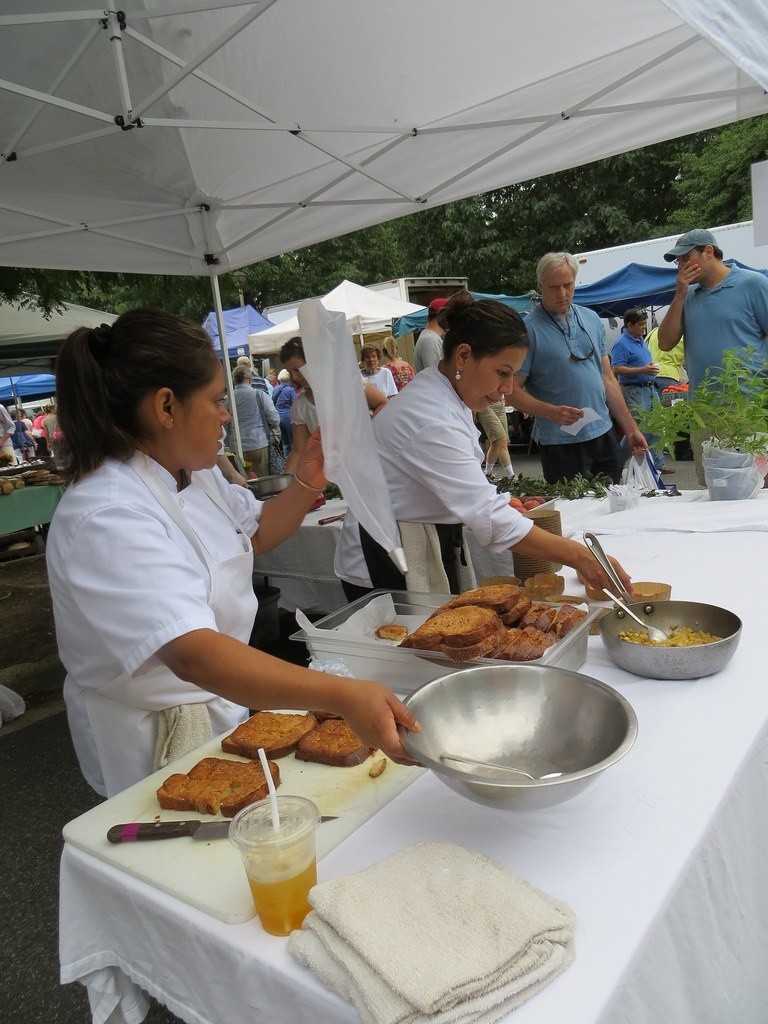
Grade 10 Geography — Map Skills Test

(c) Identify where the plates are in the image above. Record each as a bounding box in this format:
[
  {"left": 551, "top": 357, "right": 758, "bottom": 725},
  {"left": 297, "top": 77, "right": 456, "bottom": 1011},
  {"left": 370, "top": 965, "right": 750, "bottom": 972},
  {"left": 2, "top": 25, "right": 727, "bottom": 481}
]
[{"left": 509, "top": 509, "right": 564, "bottom": 583}]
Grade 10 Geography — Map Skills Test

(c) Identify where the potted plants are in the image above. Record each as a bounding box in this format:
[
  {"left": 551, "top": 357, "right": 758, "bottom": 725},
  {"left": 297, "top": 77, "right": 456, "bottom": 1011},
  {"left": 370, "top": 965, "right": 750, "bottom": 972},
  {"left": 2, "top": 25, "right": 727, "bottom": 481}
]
[{"left": 632, "top": 339, "right": 768, "bottom": 501}]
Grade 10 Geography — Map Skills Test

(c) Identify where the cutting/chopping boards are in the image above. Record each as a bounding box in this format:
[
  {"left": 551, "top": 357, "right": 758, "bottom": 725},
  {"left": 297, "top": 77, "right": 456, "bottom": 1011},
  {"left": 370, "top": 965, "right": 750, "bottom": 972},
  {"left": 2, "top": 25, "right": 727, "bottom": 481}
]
[{"left": 61, "top": 710, "right": 428, "bottom": 925}]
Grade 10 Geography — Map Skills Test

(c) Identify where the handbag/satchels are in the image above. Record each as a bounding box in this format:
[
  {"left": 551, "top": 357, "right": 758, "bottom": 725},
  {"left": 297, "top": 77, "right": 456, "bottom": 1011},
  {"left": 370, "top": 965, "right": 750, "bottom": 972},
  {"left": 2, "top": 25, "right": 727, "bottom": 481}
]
[
  {"left": 17, "top": 432, "right": 34, "bottom": 446},
  {"left": 31, "top": 429, "right": 43, "bottom": 439},
  {"left": 54, "top": 429, "right": 63, "bottom": 442},
  {"left": 626, "top": 450, "right": 667, "bottom": 490},
  {"left": 269, "top": 442, "right": 287, "bottom": 476}
]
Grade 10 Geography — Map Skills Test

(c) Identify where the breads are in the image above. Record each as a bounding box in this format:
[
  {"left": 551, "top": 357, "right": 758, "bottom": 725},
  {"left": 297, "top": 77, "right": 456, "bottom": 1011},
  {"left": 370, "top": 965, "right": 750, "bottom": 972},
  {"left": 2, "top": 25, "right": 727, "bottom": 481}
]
[
  {"left": 156, "top": 709, "right": 388, "bottom": 818},
  {"left": 0, "top": 469, "right": 64, "bottom": 494},
  {"left": 376, "top": 584, "right": 588, "bottom": 662}
]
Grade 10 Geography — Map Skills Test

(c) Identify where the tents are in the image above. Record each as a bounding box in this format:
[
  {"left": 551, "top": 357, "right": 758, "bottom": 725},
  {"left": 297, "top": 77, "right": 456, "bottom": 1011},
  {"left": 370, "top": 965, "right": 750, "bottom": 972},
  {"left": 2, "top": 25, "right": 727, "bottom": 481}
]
[
  {"left": 247, "top": 279, "right": 428, "bottom": 373},
  {"left": 531, "top": 259, "right": 768, "bottom": 342},
  {"left": 391, "top": 289, "right": 547, "bottom": 339},
  {"left": 199, "top": 303, "right": 276, "bottom": 362},
  {"left": 0, "top": 289, "right": 125, "bottom": 462}
]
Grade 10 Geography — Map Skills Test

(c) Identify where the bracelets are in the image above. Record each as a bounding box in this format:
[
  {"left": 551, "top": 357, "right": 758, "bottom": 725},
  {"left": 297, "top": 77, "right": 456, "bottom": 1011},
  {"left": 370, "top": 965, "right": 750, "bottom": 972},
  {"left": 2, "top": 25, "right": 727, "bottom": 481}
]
[{"left": 293, "top": 473, "right": 328, "bottom": 492}]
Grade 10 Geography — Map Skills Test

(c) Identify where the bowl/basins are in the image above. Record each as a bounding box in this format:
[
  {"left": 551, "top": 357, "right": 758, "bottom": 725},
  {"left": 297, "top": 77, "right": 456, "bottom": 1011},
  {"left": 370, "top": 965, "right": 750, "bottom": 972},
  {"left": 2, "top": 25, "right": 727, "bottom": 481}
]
[
  {"left": 480, "top": 573, "right": 622, "bottom": 636},
  {"left": 397, "top": 663, "right": 640, "bottom": 809},
  {"left": 623, "top": 581, "right": 671, "bottom": 601}
]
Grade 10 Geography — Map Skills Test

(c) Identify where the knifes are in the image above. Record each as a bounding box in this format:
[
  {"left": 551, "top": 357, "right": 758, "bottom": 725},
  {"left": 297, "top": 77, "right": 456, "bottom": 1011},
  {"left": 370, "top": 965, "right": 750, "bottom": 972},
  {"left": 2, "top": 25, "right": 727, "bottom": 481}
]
[{"left": 106, "top": 816, "right": 339, "bottom": 841}]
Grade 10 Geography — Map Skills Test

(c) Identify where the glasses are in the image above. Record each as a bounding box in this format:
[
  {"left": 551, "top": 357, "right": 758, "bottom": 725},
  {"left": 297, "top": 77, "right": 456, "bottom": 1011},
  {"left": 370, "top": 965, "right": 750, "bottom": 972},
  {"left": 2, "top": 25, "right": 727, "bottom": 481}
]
[
  {"left": 564, "top": 328, "right": 595, "bottom": 362},
  {"left": 636, "top": 308, "right": 647, "bottom": 317},
  {"left": 278, "top": 341, "right": 300, "bottom": 352},
  {"left": 673, "top": 249, "right": 696, "bottom": 265}
]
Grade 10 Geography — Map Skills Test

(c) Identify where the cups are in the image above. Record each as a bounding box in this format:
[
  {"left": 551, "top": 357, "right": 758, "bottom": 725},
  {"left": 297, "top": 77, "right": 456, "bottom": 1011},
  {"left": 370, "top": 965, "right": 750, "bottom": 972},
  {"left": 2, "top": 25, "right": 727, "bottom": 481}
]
[
  {"left": 607, "top": 491, "right": 640, "bottom": 512},
  {"left": 227, "top": 795, "right": 321, "bottom": 937}
]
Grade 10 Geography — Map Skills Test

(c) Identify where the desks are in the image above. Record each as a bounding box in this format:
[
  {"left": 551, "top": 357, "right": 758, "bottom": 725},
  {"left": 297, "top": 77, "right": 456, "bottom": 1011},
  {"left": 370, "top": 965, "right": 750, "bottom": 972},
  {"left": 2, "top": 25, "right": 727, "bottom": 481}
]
[{"left": 61, "top": 489, "right": 768, "bottom": 1024}]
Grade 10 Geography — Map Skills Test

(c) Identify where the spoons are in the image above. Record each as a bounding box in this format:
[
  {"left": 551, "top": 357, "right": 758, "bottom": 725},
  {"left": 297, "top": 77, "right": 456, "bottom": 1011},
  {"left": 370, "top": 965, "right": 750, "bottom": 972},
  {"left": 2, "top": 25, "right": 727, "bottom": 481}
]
[{"left": 441, "top": 753, "right": 572, "bottom": 782}]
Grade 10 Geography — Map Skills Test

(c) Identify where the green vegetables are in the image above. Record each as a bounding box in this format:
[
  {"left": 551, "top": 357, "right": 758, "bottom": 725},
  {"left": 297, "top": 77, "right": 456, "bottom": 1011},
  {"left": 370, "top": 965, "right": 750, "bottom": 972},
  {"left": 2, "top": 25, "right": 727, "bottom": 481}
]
[
  {"left": 640, "top": 487, "right": 683, "bottom": 498},
  {"left": 322, "top": 482, "right": 344, "bottom": 500},
  {"left": 497, "top": 473, "right": 607, "bottom": 500}
]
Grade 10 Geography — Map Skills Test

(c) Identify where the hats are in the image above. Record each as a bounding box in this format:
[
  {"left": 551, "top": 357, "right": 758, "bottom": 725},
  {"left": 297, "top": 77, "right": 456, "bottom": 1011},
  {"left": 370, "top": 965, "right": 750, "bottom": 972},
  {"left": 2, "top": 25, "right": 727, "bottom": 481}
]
[
  {"left": 428, "top": 298, "right": 449, "bottom": 314},
  {"left": 663, "top": 229, "right": 723, "bottom": 263}
]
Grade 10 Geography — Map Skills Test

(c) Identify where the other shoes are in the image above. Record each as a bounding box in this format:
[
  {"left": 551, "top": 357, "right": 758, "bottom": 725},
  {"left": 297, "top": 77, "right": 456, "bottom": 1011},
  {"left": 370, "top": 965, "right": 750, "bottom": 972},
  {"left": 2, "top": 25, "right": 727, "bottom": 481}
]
[
  {"left": 0, "top": 591, "right": 11, "bottom": 600},
  {"left": 481, "top": 461, "right": 486, "bottom": 467},
  {"left": 659, "top": 465, "right": 676, "bottom": 474},
  {"left": 485, "top": 469, "right": 502, "bottom": 482}
]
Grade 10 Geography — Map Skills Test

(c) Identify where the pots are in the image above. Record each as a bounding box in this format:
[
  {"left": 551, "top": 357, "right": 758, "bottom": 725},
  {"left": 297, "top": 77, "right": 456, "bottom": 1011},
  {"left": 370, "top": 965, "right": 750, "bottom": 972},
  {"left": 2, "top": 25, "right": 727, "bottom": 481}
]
[
  {"left": 246, "top": 472, "right": 293, "bottom": 500},
  {"left": 583, "top": 531, "right": 743, "bottom": 680}
]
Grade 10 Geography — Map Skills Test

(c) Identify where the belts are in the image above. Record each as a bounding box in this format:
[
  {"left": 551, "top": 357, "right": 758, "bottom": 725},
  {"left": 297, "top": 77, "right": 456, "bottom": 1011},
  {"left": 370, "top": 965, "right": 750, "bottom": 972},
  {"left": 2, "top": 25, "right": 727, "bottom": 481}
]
[{"left": 623, "top": 382, "right": 653, "bottom": 388}]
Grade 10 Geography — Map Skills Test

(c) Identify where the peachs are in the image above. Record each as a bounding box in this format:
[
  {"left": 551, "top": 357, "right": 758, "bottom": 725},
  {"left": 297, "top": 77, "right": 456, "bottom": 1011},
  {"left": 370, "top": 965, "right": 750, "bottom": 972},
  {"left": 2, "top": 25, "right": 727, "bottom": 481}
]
[{"left": 509, "top": 496, "right": 546, "bottom": 512}]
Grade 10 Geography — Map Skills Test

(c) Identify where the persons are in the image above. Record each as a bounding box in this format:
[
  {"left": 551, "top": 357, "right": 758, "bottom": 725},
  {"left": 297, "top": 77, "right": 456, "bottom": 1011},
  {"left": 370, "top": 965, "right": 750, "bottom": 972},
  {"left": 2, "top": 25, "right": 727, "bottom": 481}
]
[
  {"left": 45, "top": 306, "right": 430, "bottom": 809},
  {"left": 656, "top": 229, "right": 768, "bottom": 488},
  {"left": 608, "top": 308, "right": 690, "bottom": 473},
  {"left": 218, "top": 355, "right": 297, "bottom": 490},
  {"left": 0, "top": 402, "right": 62, "bottom": 468},
  {"left": 332, "top": 288, "right": 638, "bottom": 613},
  {"left": 277, "top": 335, "right": 389, "bottom": 501},
  {"left": 359, "top": 298, "right": 519, "bottom": 485},
  {"left": 503, "top": 251, "right": 649, "bottom": 489}
]
